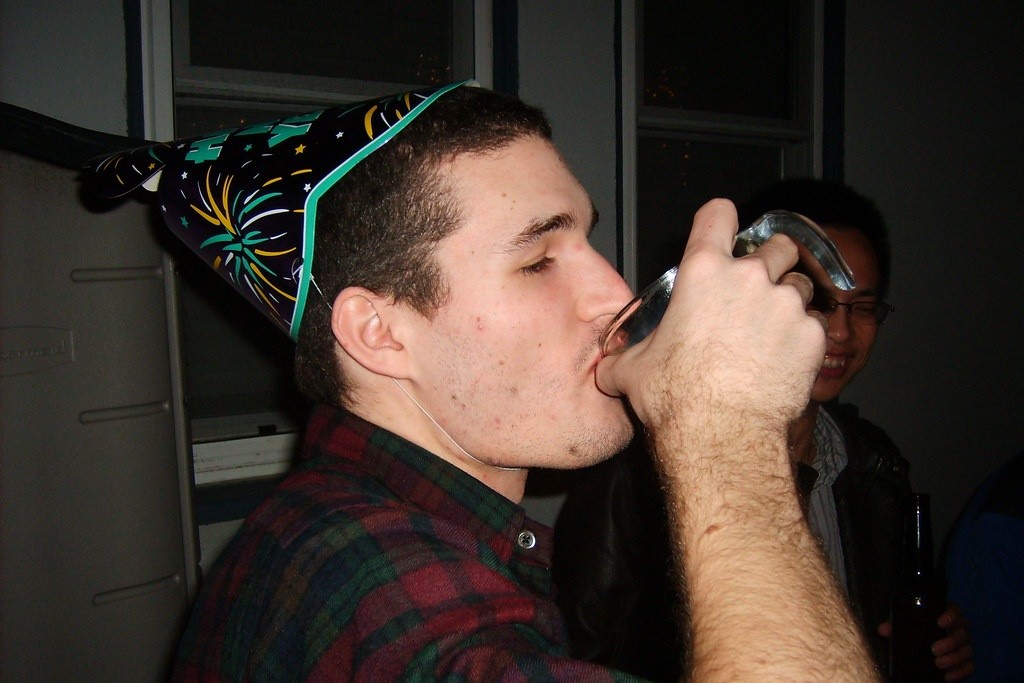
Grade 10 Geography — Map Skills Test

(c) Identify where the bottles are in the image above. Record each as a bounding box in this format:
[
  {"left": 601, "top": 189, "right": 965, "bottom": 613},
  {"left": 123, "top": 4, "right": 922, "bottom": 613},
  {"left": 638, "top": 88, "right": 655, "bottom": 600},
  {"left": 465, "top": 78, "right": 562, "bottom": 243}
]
[{"left": 890, "top": 493, "right": 948, "bottom": 683}]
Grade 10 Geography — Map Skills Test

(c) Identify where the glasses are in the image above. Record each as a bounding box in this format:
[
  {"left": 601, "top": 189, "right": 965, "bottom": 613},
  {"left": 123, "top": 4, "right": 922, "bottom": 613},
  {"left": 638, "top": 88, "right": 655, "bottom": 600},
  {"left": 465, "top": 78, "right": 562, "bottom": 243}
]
[{"left": 805, "top": 299, "right": 897, "bottom": 326}]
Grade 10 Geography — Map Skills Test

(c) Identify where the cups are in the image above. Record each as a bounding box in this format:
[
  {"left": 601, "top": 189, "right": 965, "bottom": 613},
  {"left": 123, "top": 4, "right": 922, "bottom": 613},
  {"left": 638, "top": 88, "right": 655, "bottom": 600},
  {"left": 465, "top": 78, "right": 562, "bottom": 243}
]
[{"left": 599, "top": 210, "right": 856, "bottom": 357}]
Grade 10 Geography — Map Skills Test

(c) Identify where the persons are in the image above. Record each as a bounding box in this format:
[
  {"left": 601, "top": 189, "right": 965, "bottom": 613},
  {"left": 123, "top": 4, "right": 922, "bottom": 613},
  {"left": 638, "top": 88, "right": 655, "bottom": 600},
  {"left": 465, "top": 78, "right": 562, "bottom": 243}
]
[
  {"left": 554, "top": 174, "right": 974, "bottom": 683},
  {"left": 81, "top": 77, "right": 882, "bottom": 683}
]
